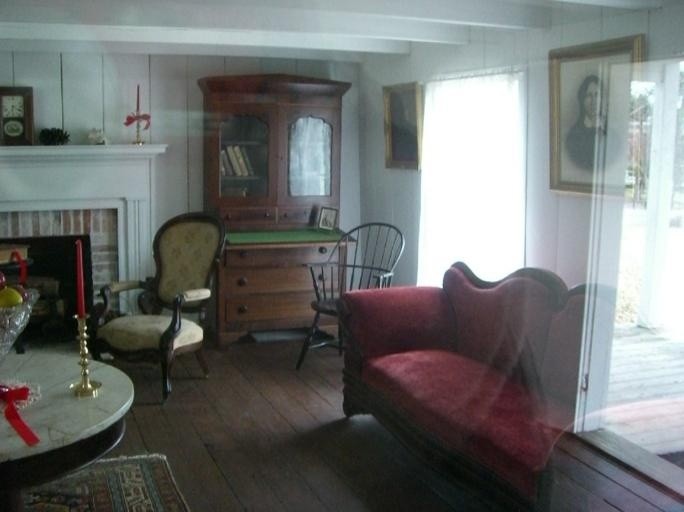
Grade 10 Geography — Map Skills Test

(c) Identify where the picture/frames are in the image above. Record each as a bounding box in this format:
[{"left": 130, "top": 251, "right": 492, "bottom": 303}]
[
  {"left": 382, "top": 82, "right": 420, "bottom": 168},
  {"left": 318, "top": 206, "right": 339, "bottom": 232},
  {"left": 549, "top": 34, "right": 647, "bottom": 200}
]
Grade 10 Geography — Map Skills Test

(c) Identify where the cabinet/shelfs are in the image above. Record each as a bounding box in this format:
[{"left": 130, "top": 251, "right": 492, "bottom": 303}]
[
  {"left": 196, "top": 72, "right": 352, "bottom": 231},
  {"left": 206, "top": 231, "right": 356, "bottom": 344}
]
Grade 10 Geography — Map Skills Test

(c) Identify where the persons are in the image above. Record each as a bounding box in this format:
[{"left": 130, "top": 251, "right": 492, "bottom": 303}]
[{"left": 563, "top": 74, "right": 624, "bottom": 173}]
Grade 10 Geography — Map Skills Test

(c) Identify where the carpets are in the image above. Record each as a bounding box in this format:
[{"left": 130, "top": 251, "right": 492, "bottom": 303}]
[{"left": 0, "top": 452, "right": 189, "bottom": 512}]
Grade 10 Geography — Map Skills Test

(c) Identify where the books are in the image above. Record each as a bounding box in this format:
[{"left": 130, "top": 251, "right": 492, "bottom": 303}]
[
  {"left": 219, "top": 145, "right": 255, "bottom": 198},
  {"left": 1, "top": 242, "right": 30, "bottom": 265}
]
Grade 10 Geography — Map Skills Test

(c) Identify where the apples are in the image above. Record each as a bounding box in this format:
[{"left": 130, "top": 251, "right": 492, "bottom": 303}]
[
  {"left": 0, "top": 287, "right": 23, "bottom": 307},
  {"left": 0, "top": 272, "right": 6, "bottom": 290},
  {"left": 9, "top": 284, "right": 29, "bottom": 302}
]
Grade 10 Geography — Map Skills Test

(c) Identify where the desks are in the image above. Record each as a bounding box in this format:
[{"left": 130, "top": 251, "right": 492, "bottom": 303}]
[{"left": 0, "top": 351, "right": 134, "bottom": 512}]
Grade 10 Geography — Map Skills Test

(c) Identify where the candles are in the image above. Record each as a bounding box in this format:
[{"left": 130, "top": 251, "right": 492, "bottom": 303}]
[
  {"left": 137, "top": 85, "right": 140, "bottom": 110},
  {"left": 75, "top": 240, "right": 86, "bottom": 317}
]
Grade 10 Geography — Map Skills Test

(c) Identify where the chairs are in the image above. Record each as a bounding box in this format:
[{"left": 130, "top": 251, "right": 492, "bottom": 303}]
[
  {"left": 88, "top": 212, "right": 227, "bottom": 405},
  {"left": 296, "top": 221, "right": 406, "bottom": 370}
]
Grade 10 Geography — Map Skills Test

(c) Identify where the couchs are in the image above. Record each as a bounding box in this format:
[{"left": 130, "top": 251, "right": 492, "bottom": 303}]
[{"left": 337, "top": 263, "right": 617, "bottom": 512}]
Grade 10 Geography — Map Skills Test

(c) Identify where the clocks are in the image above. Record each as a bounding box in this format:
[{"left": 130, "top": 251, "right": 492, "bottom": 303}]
[{"left": 0, "top": 86, "right": 33, "bottom": 146}]
